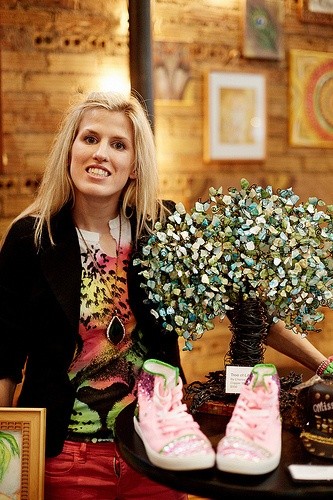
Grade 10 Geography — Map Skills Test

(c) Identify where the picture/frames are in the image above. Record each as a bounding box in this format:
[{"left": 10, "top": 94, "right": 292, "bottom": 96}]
[
  {"left": 202, "top": 67, "right": 268, "bottom": 166},
  {"left": 0, "top": 409, "right": 46, "bottom": 500},
  {"left": 242, "top": 1, "right": 286, "bottom": 64},
  {"left": 289, "top": 47, "right": 333, "bottom": 150},
  {"left": 295, "top": 0, "right": 333, "bottom": 27}
]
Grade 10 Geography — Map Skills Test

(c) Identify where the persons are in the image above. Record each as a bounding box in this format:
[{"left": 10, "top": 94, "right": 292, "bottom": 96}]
[{"left": 1, "top": 92, "right": 333, "bottom": 500}]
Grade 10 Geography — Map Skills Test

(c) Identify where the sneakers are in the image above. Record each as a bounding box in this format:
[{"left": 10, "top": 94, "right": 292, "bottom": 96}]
[
  {"left": 216, "top": 363, "right": 281, "bottom": 475},
  {"left": 133, "top": 359, "right": 216, "bottom": 470}
]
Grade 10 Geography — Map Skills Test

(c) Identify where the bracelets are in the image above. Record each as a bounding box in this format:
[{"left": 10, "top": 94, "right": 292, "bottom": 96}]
[{"left": 316, "top": 355, "right": 333, "bottom": 381}]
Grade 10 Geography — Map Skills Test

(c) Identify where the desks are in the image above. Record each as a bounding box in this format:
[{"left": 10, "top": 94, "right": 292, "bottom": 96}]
[{"left": 111, "top": 377, "right": 333, "bottom": 500}]
